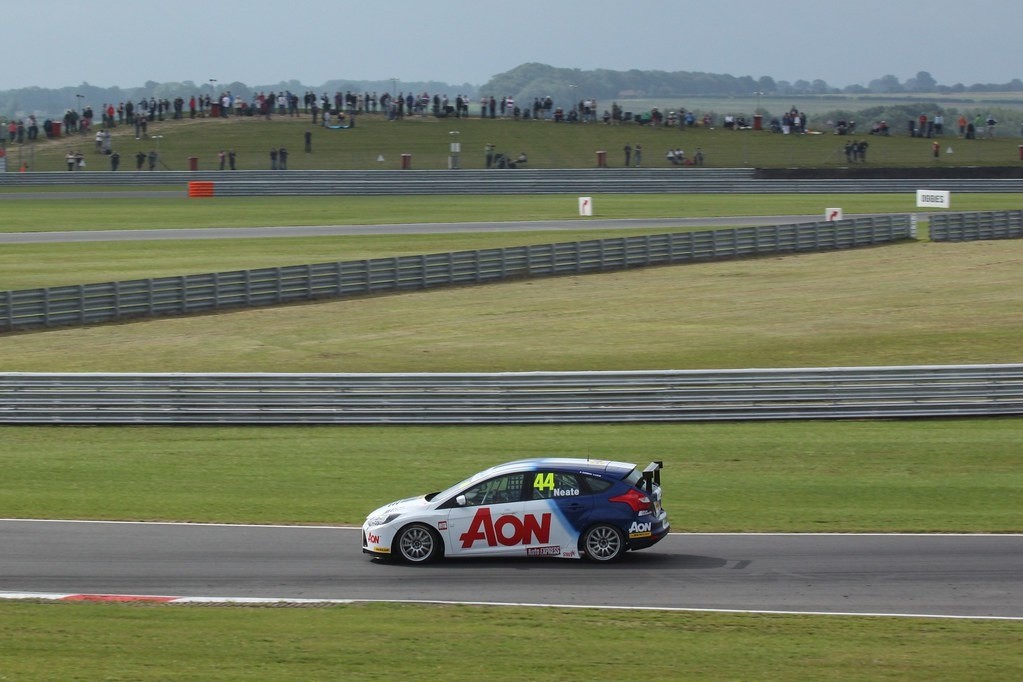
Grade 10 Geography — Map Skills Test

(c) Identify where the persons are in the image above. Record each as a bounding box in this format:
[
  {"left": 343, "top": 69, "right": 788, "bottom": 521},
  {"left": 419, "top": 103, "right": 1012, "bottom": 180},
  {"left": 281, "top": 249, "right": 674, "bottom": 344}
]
[{"left": 0, "top": 90, "right": 998, "bottom": 171}]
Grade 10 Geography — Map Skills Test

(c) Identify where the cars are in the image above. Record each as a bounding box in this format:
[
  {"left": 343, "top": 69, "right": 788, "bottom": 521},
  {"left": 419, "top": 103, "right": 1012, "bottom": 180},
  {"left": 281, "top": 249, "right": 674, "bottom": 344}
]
[{"left": 360, "top": 457, "right": 672, "bottom": 566}]
